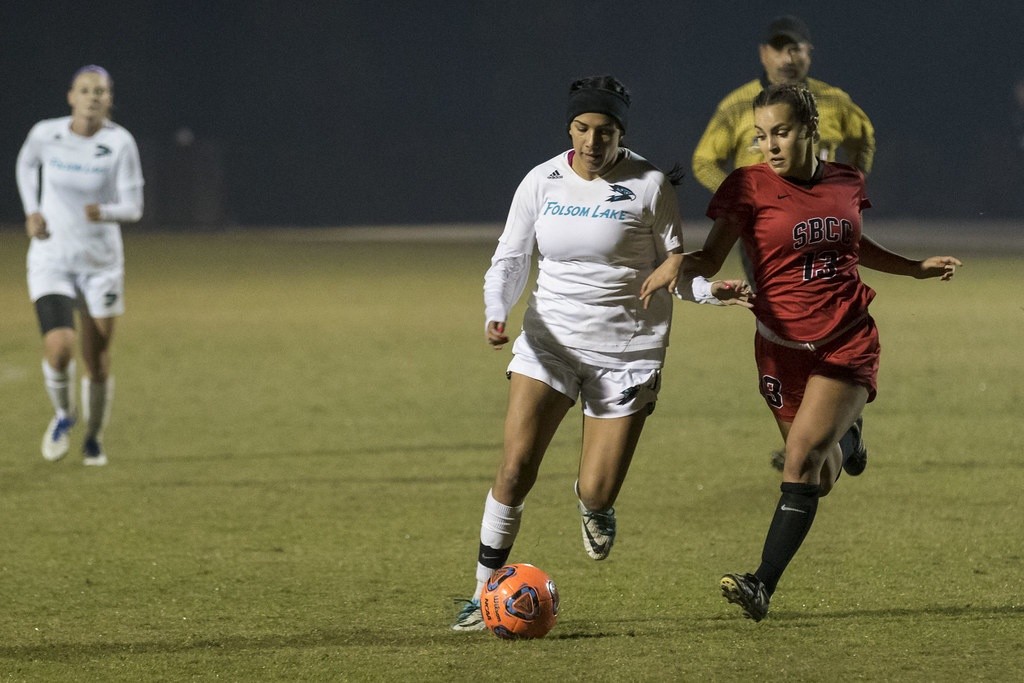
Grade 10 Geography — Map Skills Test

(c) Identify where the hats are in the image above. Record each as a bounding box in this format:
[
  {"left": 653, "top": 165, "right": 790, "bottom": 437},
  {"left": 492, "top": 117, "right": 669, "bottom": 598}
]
[
  {"left": 566, "top": 74, "right": 632, "bottom": 136},
  {"left": 761, "top": 14, "right": 811, "bottom": 44}
]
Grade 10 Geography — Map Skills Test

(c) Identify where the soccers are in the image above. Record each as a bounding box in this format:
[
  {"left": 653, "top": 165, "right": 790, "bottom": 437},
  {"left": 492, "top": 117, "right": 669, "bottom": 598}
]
[{"left": 480, "top": 563, "right": 560, "bottom": 639}]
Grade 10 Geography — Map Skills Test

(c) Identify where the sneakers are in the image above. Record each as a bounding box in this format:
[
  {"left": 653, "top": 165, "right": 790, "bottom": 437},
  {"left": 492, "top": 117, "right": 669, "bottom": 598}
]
[
  {"left": 720, "top": 572, "right": 770, "bottom": 623},
  {"left": 842, "top": 415, "right": 867, "bottom": 476},
  {"left": 574, "top": 479, "right": 616, "bottom": 560},
  {"left": 451, "top": 597, "right": 487, "bottom": 632},
  {"left": 40, "top": 406, "right": 77, "bottom": 462},
  {"left": 83, "top": 438, "right": 108, "bottom": 466}
]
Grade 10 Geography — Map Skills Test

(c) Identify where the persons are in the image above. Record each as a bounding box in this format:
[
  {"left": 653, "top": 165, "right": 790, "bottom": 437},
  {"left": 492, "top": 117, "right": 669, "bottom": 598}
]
[
  {"left": 452, "top": 75, "right": 756, "bottom": 631},
  {"left": 691, "top": 14, "right": 875, "bottom": 470},
  {"left": 15, "top": 66, "right": 144, "bottom": 466},
  {"left": 638, "top": 83, "right": 963, "bottom": 621}
]
